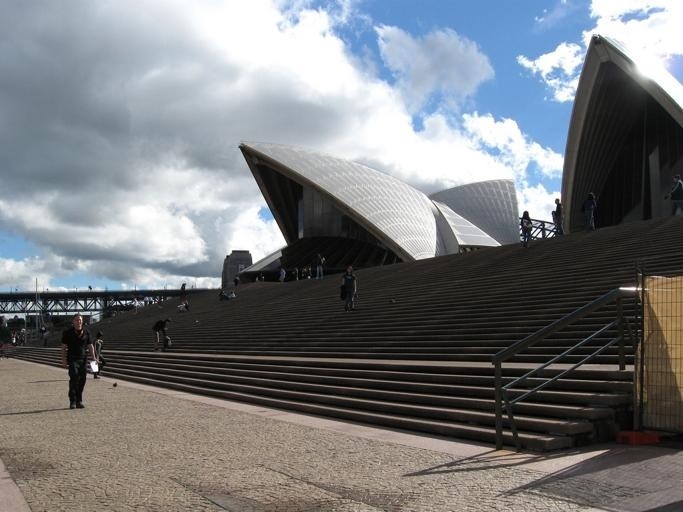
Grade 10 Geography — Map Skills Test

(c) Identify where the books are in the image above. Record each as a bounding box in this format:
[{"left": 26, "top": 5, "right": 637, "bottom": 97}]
[{"left": 90, "top": 360, "right": 99, "bottom": 372}]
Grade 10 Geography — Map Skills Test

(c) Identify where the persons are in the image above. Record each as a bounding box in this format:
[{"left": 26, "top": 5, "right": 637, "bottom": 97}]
[
  {"left": 0, "top": 283, "right": 190, "bottom": 382},
  {"left": 582, "top": 192, "right": 595, "bottom": 226},
  {"left": 217, "top": 254, "right": 324, "bottom": 302},
  {"left": 551, "top": 211, "right": 558, "bottom": 224},
  {"left": 553, "top": 198, "right": 560, "bottom": 234},
  {"left": 520, "top": 210, "right": 532, "bottom": 245},
  {"left": 340, "top": 266, "right": 358, "bottom": 312},
  {"left": 60, "top": 314, "right": 102, "bottom": 410},
  {"left": 663, "top": 174, "right": 682, "bottom": 214}
]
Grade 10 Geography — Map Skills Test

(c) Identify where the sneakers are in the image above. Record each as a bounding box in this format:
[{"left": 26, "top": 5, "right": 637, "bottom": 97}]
[
  {"left": 344, "top": 307, "right": 348, "bottom": 312},
  {"left": 350, "top": 308, "right": 358, "bottom": 313},
  {"left": 93, "top": 372, "right": 103, "bottom": 379}
]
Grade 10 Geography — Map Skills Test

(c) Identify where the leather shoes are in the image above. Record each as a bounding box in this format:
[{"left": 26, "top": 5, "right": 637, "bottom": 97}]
[
  {"left": 77, "top": 401, "right": 85, "bottom": 408},
  {"left": 70, "top": 401, "right": 76, "bottom": 409}
]
